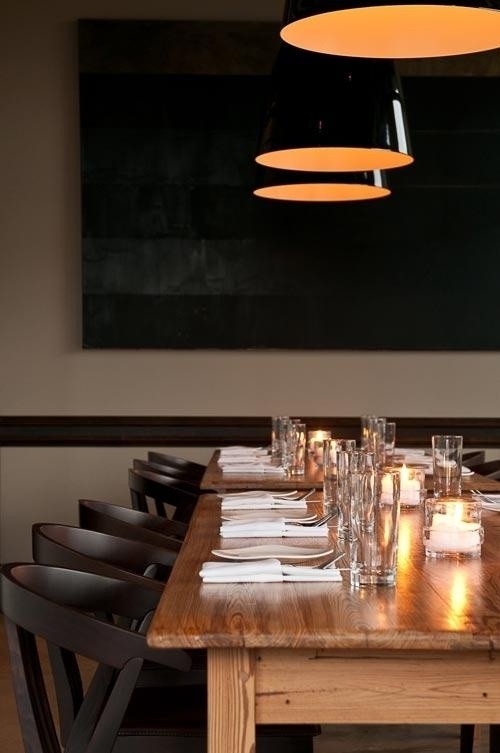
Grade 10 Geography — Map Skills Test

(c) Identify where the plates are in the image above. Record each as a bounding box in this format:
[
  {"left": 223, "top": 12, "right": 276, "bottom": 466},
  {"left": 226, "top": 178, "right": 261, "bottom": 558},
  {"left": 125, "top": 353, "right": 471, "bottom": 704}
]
[
  {"left": 215, "top": 490, "right": 300, "bottom": 499},
  {"left": 219, "top": 511, "right": 319, "bottom": 522},
  {"left": 209, "top": 544, "right": 335, "bottom": 562}
]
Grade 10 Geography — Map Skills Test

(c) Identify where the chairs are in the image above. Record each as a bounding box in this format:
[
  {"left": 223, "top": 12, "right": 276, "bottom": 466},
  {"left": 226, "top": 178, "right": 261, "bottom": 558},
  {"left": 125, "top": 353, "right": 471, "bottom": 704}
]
[{"left": 462, "top": 450, "right": 500, "bottom": 482}]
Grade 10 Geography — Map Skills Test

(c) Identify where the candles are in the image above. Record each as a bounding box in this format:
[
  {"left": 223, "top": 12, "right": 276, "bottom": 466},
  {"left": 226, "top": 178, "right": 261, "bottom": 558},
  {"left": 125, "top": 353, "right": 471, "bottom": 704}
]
[
  {"left": 383, "top": 479, "right": 420, "bottom": 506},
  {"left": 430, "top": 514, "right": 480, "bottom": 554}
]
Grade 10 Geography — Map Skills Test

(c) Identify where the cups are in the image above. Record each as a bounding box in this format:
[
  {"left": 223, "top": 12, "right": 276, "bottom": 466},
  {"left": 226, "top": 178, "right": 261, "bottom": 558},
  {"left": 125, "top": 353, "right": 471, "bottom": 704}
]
[
  {"left": 320, "top": 438, "right": 402, "bottom": 588},
  {"left": 270, "top": 414, "right": 308, "bottom": 478},
  {"left": 361, "top": 412, "right": 397, "bottom": 473},
  {"left": 431, "top": 434, "right": 463, "bottom": 500}
]
